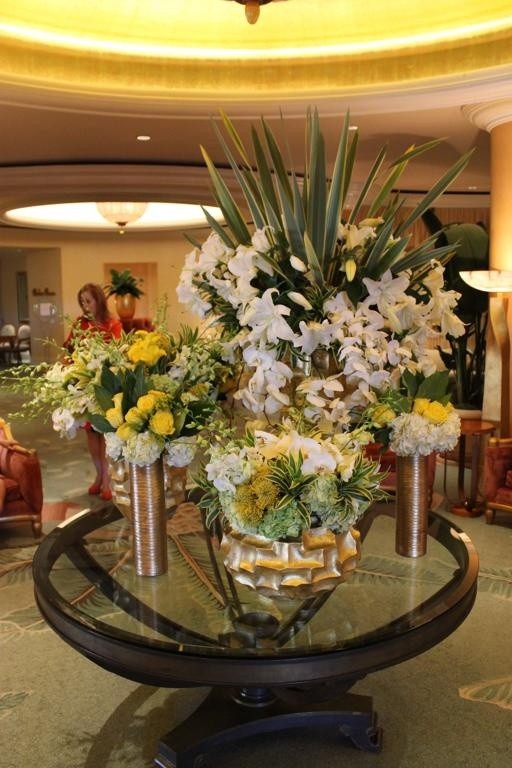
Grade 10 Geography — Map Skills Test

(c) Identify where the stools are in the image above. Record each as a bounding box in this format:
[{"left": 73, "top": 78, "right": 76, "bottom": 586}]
[{"left": 443, "top": 422, "right": 495, "bottom": 517}]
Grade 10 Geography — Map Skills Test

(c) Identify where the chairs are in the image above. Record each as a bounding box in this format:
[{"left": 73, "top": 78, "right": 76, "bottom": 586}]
[
  {"left": 0, "top": 418, "right": 43, "bottom": 540},
  {"left": 484, "top": 436, "right": 512, "bottom": 524}
]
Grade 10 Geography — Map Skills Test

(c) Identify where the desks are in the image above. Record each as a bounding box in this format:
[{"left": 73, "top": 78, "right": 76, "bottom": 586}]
[{"left": 32, "top": 483, "right": 481, "bottom": 768}]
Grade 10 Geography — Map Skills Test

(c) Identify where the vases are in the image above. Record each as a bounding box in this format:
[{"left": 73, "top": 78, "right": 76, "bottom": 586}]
[
  {"left": 395, "top": 453, "right": 431, "bottom": 558},
  {"left": 105, "top": 456, "right": 187, "bottom": 525},
  {"left": 217, "top": 527, "right": 360, "bottom": 601},
  {"left": 124, "top": 462, "right": 167, "bottom": 577}
]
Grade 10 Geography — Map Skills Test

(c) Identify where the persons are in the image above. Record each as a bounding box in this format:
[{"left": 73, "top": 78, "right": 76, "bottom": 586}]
[{"left": 58, "top": 282, "right": 124, "bottom": 501}]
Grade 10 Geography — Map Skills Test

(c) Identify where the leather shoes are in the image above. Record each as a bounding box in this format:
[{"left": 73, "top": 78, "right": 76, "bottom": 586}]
[
  {"left": 88, "top": 483, "right": 102, "bottom": 493},
  {"left": 100, "top": 486, "right": 112, "bottom": 500}
]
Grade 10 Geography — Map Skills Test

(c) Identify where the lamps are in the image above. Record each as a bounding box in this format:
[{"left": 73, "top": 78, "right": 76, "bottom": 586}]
[{"left": 95, "top": 200, "right": 147, "bottom": 231}]
